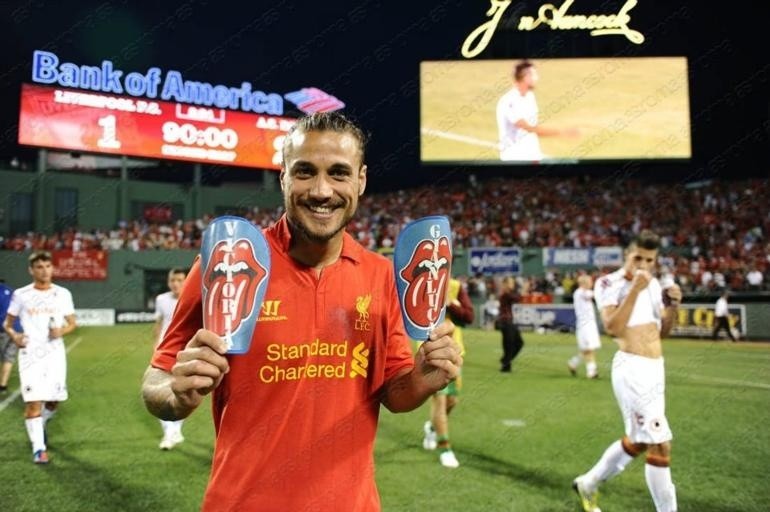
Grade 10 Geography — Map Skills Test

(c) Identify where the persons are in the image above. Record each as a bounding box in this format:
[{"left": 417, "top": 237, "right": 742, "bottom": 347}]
[
  {"left": 491, "top": 61, "right": 582, "bottom": 160},
  {"left": 565, "top": 273, "right": 606, "bottom": 378},
  {"left": 569, "top": 228, "right": 684, "bottom": 512},
  {"left": 492, "top": 273, "right": 532, "bottom": 373},
  {"left": 343, "top": 173, "right": 767, "bottom": 342},
  {"left": 142, "top": 110, "right": 466, "bottom": 512},
  {"left": 2, "top": 248, "right": 78, "bottom": 463},
  {"left": 0, "top": 206, "right": 286, "bottom": 251},
  {"left": 0, "top": 279, "right": 22, "bottom": 396},
  {"left": 416, "top": 275, "right": 472, "bottom": 472},
  {"left": 152, "top": 264, "right": 189, "bottom": 452}
]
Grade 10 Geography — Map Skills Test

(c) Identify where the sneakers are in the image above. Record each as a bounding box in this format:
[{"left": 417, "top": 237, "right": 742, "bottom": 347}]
[
  {"left": 440, "top": 451, "right": 460, "bottom": 468},
  {"left": 572, "top": 476, "right": 602, "bottom": 512},
  {"left": 159, "top": 434, "right": 176, "bottom": 450},
  {"left": 32, "top": 449, "right": 48, "bottom": 464},
  {"left": 423, "top": 420, "right": 438, "bottom": 450}
]
